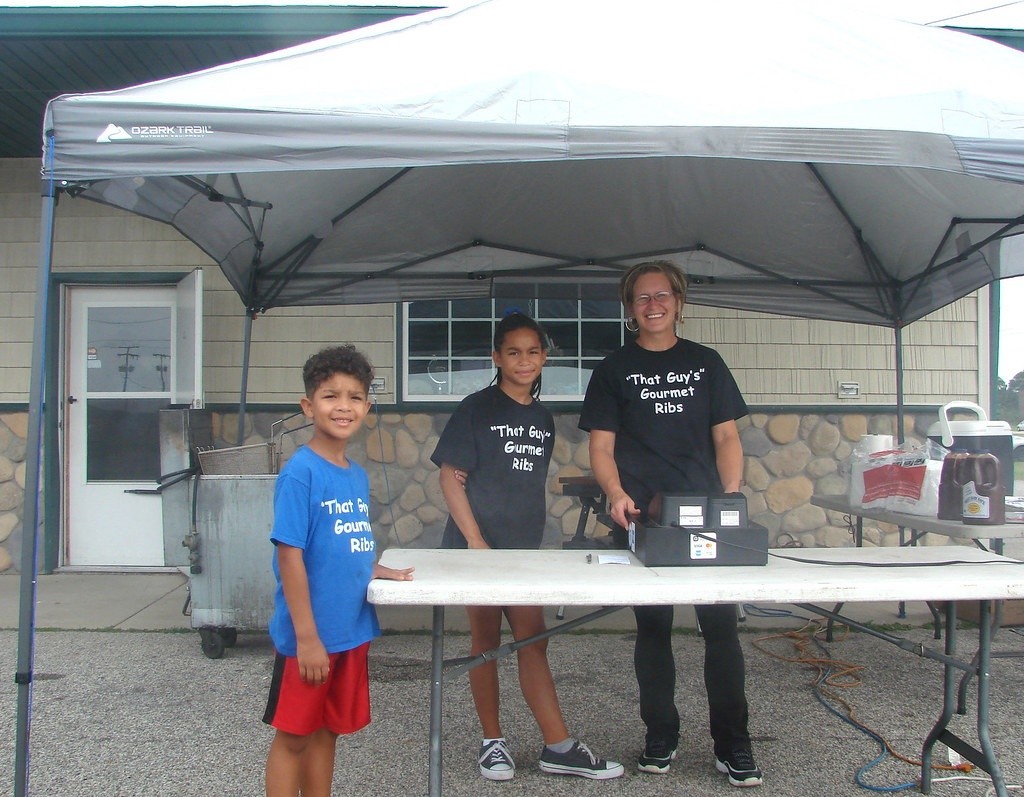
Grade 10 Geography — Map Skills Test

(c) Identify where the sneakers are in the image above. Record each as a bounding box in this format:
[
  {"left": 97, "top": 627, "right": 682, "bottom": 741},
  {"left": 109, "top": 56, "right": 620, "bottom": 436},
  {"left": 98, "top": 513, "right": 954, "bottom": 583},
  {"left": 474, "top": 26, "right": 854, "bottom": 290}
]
[
  {"left": 715, "top": 745, "right": 763, "bottom": 786},
  {"left": 478, "top": 739, "right": 516, "bottom": 781},
  {"left": 637, "top": 738, "right": 676, "bottom": 773},
  {"left": 537, "top": 738, "right": 625, "bottom": 779}
]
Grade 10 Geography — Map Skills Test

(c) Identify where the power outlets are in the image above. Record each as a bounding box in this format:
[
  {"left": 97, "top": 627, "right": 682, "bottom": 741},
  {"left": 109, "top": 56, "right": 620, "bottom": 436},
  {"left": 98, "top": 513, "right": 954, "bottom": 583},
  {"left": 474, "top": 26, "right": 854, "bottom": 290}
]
[{"left": 368, "top": 377, "right": 385, "bottom": 390}]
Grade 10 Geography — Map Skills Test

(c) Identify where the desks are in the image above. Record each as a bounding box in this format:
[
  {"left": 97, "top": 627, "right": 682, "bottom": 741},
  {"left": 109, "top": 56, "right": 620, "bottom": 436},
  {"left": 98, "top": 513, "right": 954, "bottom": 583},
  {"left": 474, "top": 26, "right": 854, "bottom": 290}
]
[
  {"left": 811, "top": 494, "right": 1024, "bottom": 716},
  {"left": 366, "top": 544, "right": 1024, "bottom": 797}
]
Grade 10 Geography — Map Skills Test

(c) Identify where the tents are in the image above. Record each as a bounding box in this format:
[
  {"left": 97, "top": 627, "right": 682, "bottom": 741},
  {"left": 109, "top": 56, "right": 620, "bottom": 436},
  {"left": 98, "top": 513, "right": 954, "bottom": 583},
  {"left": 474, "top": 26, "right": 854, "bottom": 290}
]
[{"left": 14, "top": 0, "right": 1024, "bottom": 797}]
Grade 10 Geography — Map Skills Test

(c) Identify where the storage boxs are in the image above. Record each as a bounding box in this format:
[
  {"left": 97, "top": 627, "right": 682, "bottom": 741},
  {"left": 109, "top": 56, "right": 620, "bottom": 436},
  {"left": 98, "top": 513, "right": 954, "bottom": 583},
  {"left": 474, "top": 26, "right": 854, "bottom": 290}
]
[{"left": 926, "top": 400, "right": 1014, "bottom": 496}]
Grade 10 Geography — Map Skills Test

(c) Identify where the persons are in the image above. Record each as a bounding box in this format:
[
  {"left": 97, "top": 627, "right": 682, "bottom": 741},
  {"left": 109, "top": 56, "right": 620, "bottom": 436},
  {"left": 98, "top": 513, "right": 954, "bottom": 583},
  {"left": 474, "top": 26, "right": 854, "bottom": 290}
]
[
  {"left": 578, "top": 261, "right": 762, "bottom": 786},
  {"left": 260, "top": 346, "right": 415, "bottom": 797},
  {"left": 430, "top": 314, "right": 624, "bottom": 779}
]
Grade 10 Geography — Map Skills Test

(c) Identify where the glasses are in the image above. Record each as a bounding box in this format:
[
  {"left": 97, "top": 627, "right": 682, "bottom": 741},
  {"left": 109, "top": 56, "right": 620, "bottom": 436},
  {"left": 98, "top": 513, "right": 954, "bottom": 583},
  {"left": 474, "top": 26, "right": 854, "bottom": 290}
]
[{"left": 632, "top": 292, "right": 676, "bottom": 306}]
